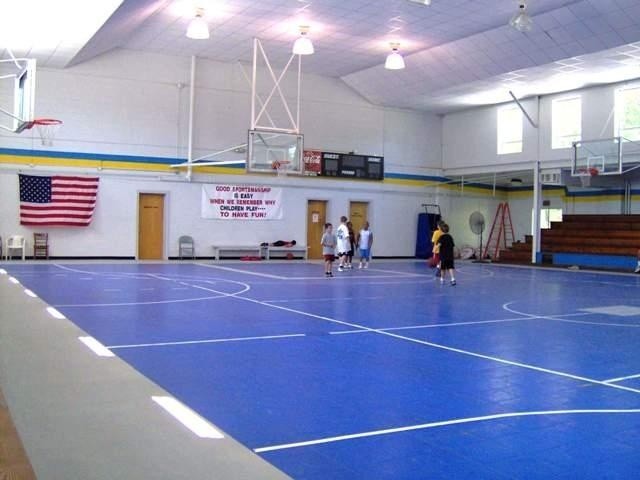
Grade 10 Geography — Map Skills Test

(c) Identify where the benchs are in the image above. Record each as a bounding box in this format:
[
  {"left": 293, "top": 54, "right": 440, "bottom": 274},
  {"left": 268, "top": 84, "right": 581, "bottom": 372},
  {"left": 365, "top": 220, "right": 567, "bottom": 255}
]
[
  {"left": 213, "top": 244, "right": 307, "bottom": 261},
  {"left": 498, "top": 214, "right": 639, "bottom": 263}
]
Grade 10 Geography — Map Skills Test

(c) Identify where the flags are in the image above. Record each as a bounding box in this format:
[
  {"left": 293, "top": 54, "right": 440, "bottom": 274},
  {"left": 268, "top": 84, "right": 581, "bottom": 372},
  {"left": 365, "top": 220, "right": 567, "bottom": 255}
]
[{"left": 18, "top": 174, "right": 100, "bottom": 227}]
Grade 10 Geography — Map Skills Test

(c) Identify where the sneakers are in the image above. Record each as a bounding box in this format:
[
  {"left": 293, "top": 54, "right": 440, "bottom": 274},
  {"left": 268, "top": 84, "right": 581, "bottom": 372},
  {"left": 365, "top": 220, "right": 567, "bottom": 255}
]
[
  {"left": 440, "top": 279, "right": 456, "bottom": 285},
  {"left": 338, "top": 263, "right": 351, "bottom": 271},
  {"left": 324, "top": 272, "right": 333, "bottom": 277},
  {"left": 359, "top": 262, "right": 368, "bottom": 268}
]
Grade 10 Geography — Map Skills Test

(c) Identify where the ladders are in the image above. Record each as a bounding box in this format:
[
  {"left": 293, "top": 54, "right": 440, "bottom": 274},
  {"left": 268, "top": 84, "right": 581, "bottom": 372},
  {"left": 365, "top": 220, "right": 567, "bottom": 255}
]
[{"left": 484, "top": 201, "right": 515, "bottom": 262}]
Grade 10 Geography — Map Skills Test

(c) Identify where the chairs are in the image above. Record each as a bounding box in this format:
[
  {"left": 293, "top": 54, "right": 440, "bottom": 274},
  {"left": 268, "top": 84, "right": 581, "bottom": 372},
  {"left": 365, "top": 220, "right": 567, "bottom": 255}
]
[
  {"left": 5, "top": 232, "right": 50, "bottom": 262},
  {"left": 178, "top": 236, "right": 195, "bottom": 260}
]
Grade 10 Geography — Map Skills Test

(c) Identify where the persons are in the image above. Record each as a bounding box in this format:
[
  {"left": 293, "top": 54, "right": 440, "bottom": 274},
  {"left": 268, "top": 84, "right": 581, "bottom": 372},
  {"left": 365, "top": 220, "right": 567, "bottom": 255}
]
[
  {"left": 337, "top": 216, "right": 352, "bottom": 271},
  {"left": 345, "top": 222, "right": 357, "bottom": 268},
  {"left": 261, "top": 239, "right": 296, "bottom": 248},
  {"left": 320, "top": 223, "right": 336, "bottom": 278},
  {"left": 431, "top": 220, "right": 446, "bottom": 278},
  {"left": 433, "top": 224, "right": 457, "bottom": 286},
  {"left": 355, "top": 222, "right": 374, "bottom": 269}
]
